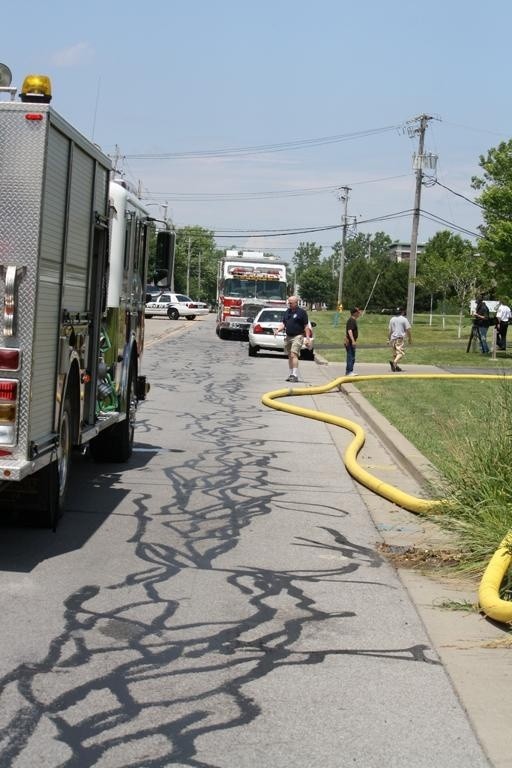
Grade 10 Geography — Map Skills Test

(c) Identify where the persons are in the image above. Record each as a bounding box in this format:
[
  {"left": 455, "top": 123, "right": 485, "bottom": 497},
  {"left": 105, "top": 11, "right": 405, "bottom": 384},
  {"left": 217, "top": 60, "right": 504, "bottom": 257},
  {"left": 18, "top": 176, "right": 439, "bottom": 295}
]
[
  {"left": 385, "top": 305, "right": 413, "bottom": 372},
  {"left": 344, "top": 307, "right": 361, "bottom": 376},
  {"left": 273, "top": 295, "right": 311, "bottom": 383},
  {"left": 496, "top": 298, "right": 511, "bottom": 350},
  {"left": 471, "top": 296, "right": 490, "bottom": 356}
]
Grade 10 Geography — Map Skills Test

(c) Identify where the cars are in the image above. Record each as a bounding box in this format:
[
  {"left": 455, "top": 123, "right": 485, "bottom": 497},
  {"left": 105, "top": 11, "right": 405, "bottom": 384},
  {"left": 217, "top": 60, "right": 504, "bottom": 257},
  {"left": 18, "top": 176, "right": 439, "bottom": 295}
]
[
  {"left": 145, "top": 289, "right": 209, "bottom": 320},
  {"left": 247, "top": 298, "right": 317, "bottom": 361}
]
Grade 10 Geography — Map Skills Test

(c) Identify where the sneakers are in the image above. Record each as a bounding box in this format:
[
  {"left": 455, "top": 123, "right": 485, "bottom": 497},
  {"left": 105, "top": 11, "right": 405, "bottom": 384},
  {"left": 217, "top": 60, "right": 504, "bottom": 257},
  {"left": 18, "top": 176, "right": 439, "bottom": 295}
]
[
  {"left": 389, "top": 360, "right": 403, "bottom": 372},
  {"left": 344, "top": 371, "right": 357, "bottom": 376}
]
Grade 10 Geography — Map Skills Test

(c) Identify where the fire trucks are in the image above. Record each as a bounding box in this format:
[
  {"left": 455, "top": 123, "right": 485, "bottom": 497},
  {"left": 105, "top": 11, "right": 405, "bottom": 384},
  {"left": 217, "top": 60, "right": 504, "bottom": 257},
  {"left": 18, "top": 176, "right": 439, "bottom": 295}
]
[
  {"left": 216, "top": 247, "right": 290, "bottom": 338},
  {"left": 0, "top": 60, "right": 177, "bottom": 526}
]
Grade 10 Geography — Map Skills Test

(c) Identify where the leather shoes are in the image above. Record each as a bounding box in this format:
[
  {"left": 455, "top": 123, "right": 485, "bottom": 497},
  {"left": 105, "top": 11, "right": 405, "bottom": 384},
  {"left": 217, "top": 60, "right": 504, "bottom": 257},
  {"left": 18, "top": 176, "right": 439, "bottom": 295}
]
[{"left": 286, "top": 374, "right": 298, "bottom": 382}]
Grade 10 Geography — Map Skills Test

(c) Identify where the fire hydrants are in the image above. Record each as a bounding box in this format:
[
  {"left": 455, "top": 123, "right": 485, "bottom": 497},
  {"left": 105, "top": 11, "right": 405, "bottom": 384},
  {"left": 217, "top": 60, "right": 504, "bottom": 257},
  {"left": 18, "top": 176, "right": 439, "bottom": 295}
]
[{"left": 339, "top": 304, "right": 343, "bottom": 312}]
